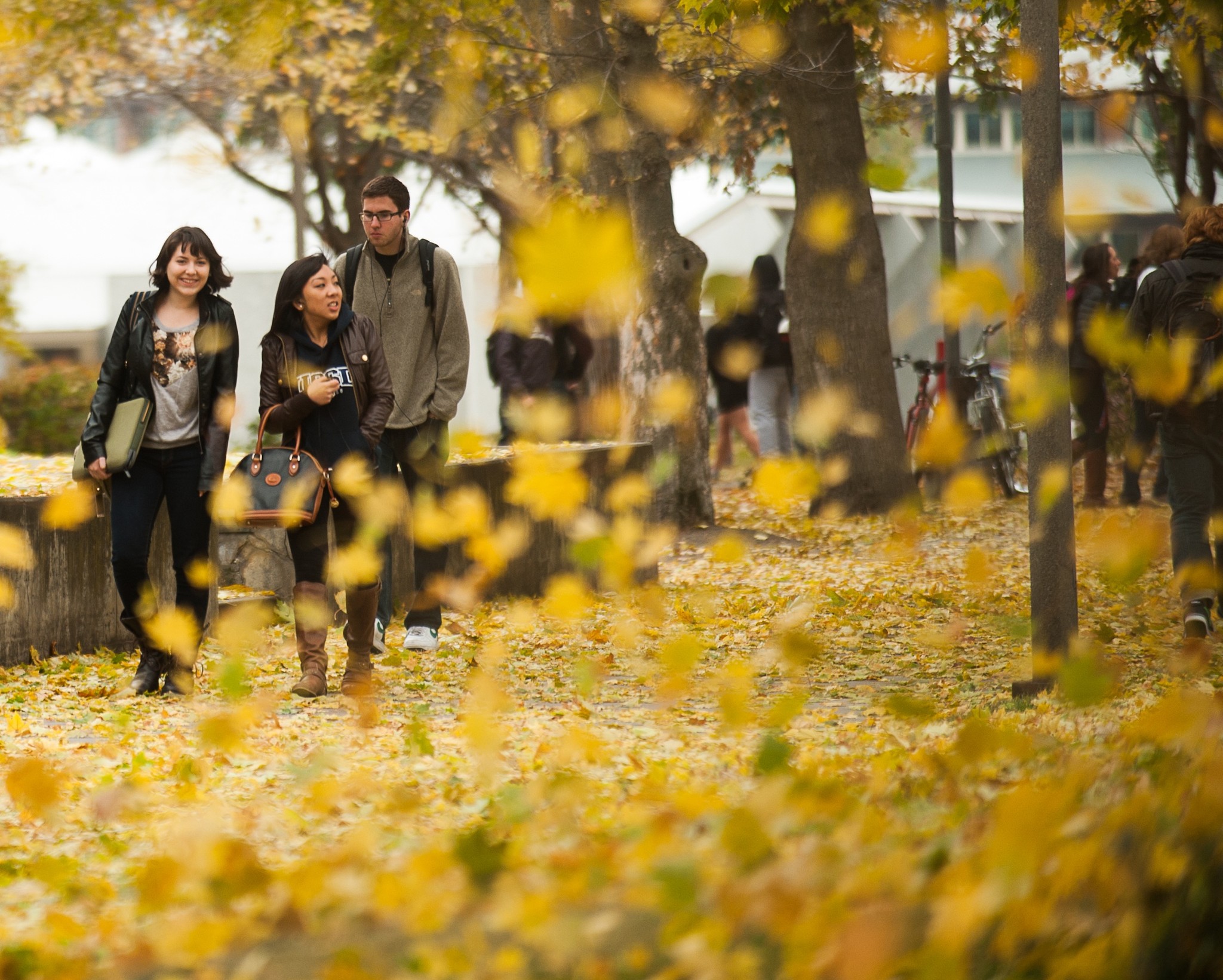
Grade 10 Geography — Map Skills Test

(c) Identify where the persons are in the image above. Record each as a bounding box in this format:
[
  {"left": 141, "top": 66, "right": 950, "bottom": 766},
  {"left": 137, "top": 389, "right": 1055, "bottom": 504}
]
[
  {"left": 736, "top": 254, "right": 792, "bottom": 460},
  {"left": 259, "top": 253, "right": 395, "bottom": 697},
  {"left": 1120, "top": 224, "right": 1185, "bottom": 505},
  {"left": 1071, "top": 243, "right": 1121, "bottom": 507},
  {"left": 80, "top": 227, "right": 239, "bottom": 694},
  {"left": 1126, "top": 206, "right": 1223, "bottom": 638},
  {"left": 332, "top": 176, "right": 470, "bottom": 650},
  {"left": 490, "top": 293, "right": 593, "bottom": 444},
  {"left": 705, "top": 294, "right": 759, "bottom": 476}
]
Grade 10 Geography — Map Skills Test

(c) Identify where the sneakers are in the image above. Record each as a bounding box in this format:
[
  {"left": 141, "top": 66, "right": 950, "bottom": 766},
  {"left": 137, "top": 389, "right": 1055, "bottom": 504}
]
[
  {"left": 403, "top": 626, "right": 439, "bottom": 651},
  {"left": 371, "top": 618, "right": 385, "bottom": 654}
]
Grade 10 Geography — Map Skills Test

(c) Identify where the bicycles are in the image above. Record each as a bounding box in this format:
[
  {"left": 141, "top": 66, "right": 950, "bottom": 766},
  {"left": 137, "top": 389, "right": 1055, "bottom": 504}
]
[
  {"left": 892, "top": 354, "right": 947, "bottom": 489},
  {"left": 947, "top": 317, "right": 1026, "bottom": 500}
]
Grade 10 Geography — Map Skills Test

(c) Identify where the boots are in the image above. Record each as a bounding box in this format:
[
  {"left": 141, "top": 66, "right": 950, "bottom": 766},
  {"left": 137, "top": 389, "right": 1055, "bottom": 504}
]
[
  {"left": 120, "top": 608, "right": 169, "bottom": 695},
  {"left": 291, "top": 582, "right": 327, "bottom": 697},
  {"left": 160, "top": 622, "right": 211, "bottom": 695},
  {"left": 342, "top": 577, "right": 383, "bottom": 696}
]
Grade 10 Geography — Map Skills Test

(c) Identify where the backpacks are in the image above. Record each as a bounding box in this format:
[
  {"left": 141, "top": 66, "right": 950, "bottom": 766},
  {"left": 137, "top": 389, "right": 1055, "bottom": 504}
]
[{"left": 1160, "top": 259, "right": 1223, "bottom": 405}]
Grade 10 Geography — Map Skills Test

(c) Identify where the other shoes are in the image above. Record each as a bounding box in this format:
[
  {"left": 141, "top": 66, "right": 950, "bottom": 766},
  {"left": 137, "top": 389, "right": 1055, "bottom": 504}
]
[
  {"left": 1182, "top": 602, "right": 1213, "bottom": 640},
  {"left": 1153, "top": 485, "right": 1168, "bottom": 498},
  {"left": 1121, "top": 486, "right": 1142, "bottom": 504},
  {"left": 1083, "top": 493, "right": 1109, "bottom": 508}
]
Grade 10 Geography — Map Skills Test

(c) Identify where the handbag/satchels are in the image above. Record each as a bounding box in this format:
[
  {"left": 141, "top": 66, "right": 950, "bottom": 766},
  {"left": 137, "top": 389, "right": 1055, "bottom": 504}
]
[{"left": 232, "top": 404, "right": 340, "bottom": 528}]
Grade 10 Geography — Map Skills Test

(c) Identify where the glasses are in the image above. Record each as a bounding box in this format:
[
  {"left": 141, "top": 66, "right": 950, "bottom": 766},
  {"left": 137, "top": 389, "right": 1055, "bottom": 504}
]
[{"left": 358, "top": 210, "right": 401, "bottom": 222}]
